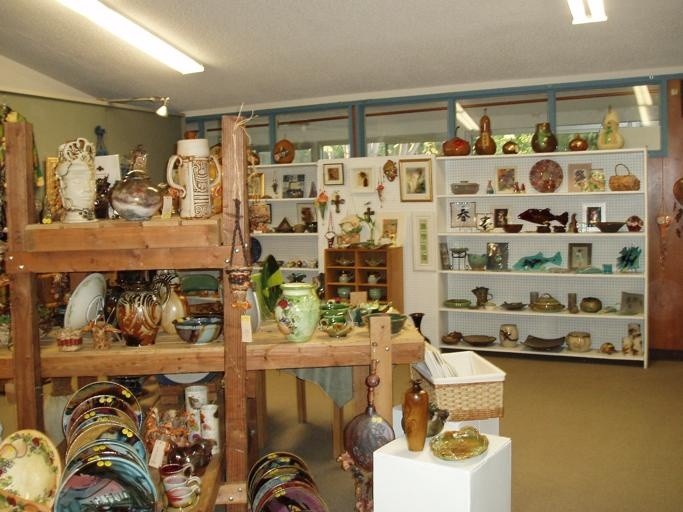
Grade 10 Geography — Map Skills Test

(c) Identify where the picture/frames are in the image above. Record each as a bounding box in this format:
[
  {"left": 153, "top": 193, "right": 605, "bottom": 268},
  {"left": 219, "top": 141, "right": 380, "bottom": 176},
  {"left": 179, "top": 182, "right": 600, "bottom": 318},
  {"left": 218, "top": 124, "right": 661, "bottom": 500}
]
[
  {"left": 322, "top": 163, "right": 347, "bottom": 187},
  {"left": 568, "top": 243, "right": 593, "bottom": 272},
  {"left": 581, "top": 202, "right": 606, "bottom": 233},
  {"left": 398, "top": 159, "right": 434, "bottom": 202},
  {"left": 568, "top": 163, "right": 593, "bottom": 193},
  {"left": 376, "top": 213, "right": 405, "bottom": 245},
  {"left": 412, "top": 211, "right": 439, "bottom": 272},
  {"left": 495, "top": 167, "right": 517, "bottom": 193},
  {"left": 296, "top": 202, "right": 319, "bottom": 225},
  {"left": 449, "top": 201, "right": 478, "bottom": 229},
  {"left": 490, "top": 205, "right": 511, "bottom": 231},
  {"left": 248, "top": 172, "right": 264, "bottom": 199},
  {"left": 350, "top": 167, "right": 375, "bottom": 195}
]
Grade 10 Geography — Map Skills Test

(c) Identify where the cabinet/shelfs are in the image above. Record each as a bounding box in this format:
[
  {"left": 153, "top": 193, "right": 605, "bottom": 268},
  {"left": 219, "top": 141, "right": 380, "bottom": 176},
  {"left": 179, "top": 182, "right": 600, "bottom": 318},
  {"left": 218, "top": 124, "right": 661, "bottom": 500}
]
[
  {"left": 372, "top": 431, "right": 512, "bottom": 512},
  {"left": 325, "top": 246, "right": 404, "bottom": 317},
  {"left": 0, "top": 113, "right": 394, "bottom": 512},
  {"left": 437, "top": 148, "right": 648, "bottom": 370},
  {"left": 246, "top": 162, "right": 320, "bottom": 287}
]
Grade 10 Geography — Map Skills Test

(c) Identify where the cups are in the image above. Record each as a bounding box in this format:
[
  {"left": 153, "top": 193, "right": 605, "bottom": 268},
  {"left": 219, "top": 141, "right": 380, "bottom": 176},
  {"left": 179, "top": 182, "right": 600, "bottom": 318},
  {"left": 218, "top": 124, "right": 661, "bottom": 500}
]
[
  {"left": 198, "top": 403, "right": 221, "bottom": 457},
  {"left": 160, "top": 462, "right": 202, "bottom": 508},
  {"left": 316, "top": 300, "right": 405, "bottom": 336},
  {"left": 184, "top": 384, "right": 207, "bottom": 444},
  {"left": 166, "top": 136, "right": 221, "bottom": 221},
  {"left": 568, "top": 293, "right": 576, "bottom": 308},
  {"left": 529, "top": 292, "right": 538, "bottom": 306},
  {"left": 602, "top": 264, "right": 613, "bottom": 274}
]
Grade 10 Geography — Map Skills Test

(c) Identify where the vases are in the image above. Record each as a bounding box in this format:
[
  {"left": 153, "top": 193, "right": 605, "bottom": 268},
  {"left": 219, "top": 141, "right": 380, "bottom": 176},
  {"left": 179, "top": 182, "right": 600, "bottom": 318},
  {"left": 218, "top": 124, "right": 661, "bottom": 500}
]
[
  {"left": 565, "top": 331, "right": 593, "bottom": 354},
  {"left": 411, "top": 312, "right": 429, "bottom": 348},
  {"left": 498, "top": 323, "right": 519, "bottom": 351},
  {"left": 532, "top": 122, "right": 559, "bottom": 155},
  {"left": 579, "top": 298, "right": 603, "bottom": 314},
  {"left": 401, "top": 379, "right": 429, "bottom": 452},
  {"left": 110, "top": 147, "right": 163, "bottom": 223},
  {"left": 55, "top": 138, "right": 102, "bottom": 226},
  {"left": 276, "top": 282, "right": 319, "bottom": 342}
]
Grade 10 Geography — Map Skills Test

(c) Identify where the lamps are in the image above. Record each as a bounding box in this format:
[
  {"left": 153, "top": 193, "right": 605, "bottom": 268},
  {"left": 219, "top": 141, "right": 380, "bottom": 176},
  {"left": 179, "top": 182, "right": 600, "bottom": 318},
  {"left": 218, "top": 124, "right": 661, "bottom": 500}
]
[{"left": 103, "top": 96, "right": 174, "bottom": 118}]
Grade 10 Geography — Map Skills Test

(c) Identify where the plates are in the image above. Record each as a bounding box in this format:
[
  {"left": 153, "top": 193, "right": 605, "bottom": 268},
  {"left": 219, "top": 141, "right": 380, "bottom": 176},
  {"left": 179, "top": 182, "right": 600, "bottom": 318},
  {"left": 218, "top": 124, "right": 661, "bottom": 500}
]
[
  {"left": 64, "top": 272, "right": 106, "bottom": 331},
  {"left": 529, "top": 158, "right": 563, "bottom": 192},
  {"left": 500, "top": 303, "right": 525, "bottom": 310},
  {"left": 273, "top": 140, "right": 296, "bottom": 163},
  {"left": 246, "top": 451, "right": 330, "bottom": 511},
  {"left": 1, "top": 381, "right": 156, "bottom": 512},
  {"left": 519, "top": 335, "right": 565, "bottom": 351},
  {"left": 443, "top": 299, "right": 471, "bottom": 308},
  {"left": 251, "top": 237, "right": 262, "bottom": 267}
]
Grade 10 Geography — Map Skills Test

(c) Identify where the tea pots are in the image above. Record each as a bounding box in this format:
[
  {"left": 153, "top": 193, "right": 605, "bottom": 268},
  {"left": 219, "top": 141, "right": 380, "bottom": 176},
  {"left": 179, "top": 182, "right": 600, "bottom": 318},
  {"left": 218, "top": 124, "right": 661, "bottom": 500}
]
[
  {"left": 108, "top": 167, "right": 166, "bottom": 223},
  {"left": 472, "top": 287, "right": 492, "bottom": 306},
  {"left": 334, "top": 253, "right": 383, "bottom": 283}
]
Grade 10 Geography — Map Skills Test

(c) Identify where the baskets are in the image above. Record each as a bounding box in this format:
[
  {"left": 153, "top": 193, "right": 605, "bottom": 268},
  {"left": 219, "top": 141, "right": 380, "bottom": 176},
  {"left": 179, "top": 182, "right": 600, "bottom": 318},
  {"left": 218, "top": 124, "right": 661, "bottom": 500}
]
[{"left": 411, "top": 349, "right": 506, "bottom": 421}]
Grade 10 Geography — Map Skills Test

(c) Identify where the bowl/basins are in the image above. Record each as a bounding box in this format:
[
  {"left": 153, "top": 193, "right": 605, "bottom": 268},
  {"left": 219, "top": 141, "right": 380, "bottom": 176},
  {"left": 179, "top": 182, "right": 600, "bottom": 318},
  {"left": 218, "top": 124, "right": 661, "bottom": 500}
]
[
  {"left": 451, "top": 181, "right": 480, "bottom": 194},
  {"left": 337, "top": 287, "right": 352, "bottom": 297},
  {"left": 592, "top": 222, "right": 627, "bottom": 232},
  {"left": 170, "top": 315, "right": 221, "bottom": 342},
  {"left": 501, "top": 225, "right": 522, "bottom": 232},
  {"left": 463, "top": 335, "right": 495, "bottom": 346},
  {"left": 367, "top": 289, "right": 384, "bottom": 299}
]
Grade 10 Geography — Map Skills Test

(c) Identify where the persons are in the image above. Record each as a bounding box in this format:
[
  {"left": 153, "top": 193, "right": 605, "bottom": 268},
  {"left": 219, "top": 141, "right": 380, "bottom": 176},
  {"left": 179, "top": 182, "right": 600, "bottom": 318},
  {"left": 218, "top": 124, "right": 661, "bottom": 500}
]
[
  {"left": 496, "top": 210, "right": 505, "bottom": 225},
  {"left": 357, "top": 172, "right": 368, "bottom": 186},
  {"left": 588, "top": 208, "right": 598, "bottom": 225},
  {"left": 499, "top": 169, "right": 514, "bottom": 194},
  {"left": 405, "top": 168, "right": 424, "bottom": 194},
  {"left": 327, "top": 169, "right": 338, "bottom": 180},
  {"left": 574, "top": 249, "right": 582, "bottom": 269}
]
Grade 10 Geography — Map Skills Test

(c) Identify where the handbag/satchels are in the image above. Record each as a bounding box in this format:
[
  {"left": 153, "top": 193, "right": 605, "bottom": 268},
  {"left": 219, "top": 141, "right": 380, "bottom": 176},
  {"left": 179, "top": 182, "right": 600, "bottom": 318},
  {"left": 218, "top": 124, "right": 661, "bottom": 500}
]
[{"left": 609, "top": 164, "right": 640, "bottom": 191}]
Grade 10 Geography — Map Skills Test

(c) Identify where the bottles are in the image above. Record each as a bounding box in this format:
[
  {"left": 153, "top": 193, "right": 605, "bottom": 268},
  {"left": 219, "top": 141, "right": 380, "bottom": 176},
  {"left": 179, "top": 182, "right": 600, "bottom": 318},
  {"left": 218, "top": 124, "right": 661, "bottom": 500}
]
[{"left": 486, "top": 179, "right": 493, "bottom": 193}]
